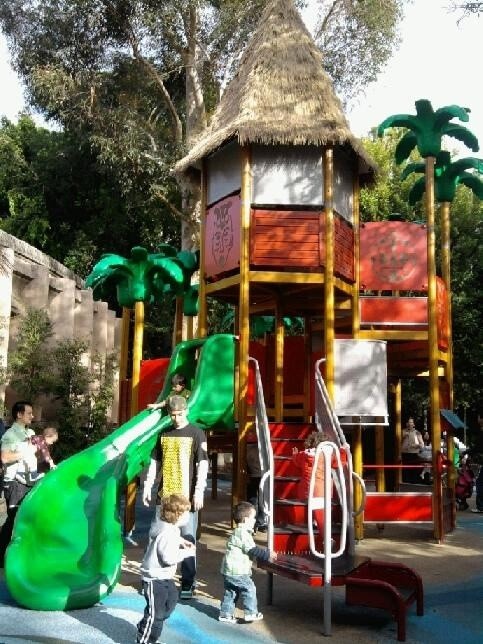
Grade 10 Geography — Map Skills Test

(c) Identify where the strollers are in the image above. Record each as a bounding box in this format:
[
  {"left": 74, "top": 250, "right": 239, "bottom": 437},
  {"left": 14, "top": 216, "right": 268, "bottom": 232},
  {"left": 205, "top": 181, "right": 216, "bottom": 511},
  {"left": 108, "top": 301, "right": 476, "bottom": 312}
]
[{"left": 441, "top": 453, "right": 474, "bottom": 511}]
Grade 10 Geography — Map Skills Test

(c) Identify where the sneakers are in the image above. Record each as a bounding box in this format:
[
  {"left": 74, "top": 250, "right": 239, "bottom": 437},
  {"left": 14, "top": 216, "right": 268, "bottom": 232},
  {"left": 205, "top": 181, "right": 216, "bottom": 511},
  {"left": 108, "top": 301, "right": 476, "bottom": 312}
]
[
  {"left": 470, "top": 508, "right": 483, "bottom": 513},
  {"left": 218, "top": 615, "right": 239, "bottom": 624},
  {"left": 178, "top": 580, "right": 196, "bottom": 601},
  {"left": 244, "top": 612, "right": 264, "bottom": 622}
]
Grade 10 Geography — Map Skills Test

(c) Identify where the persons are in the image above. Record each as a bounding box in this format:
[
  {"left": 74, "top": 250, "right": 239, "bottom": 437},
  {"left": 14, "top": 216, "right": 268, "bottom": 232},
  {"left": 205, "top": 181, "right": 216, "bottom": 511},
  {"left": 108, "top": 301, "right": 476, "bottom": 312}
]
[
  {"left": 140, "top": 394, "right": 210, "bottom": 597},
  {"left": 134, "top": 493, "right": 195, "bottom": 644},
  {"left": 1, "top": 426, "right": 60, "bottom": 489},
  {"left": 215, "top": 501, "right": 279, "bottom": 625},
  {"left": 0, "top": 399, "right": 45, "bottom": 568},
  {"left": 401, "top": 416, "right": 425, "bottom": 484},
  {"left": 417, "top": 429, "right": 483, "bottom": 514},
  {"left": 289, "top": 429, "right": 349, "bottom": 553},
  {"left": 146, "top": 373, "right": 191, "bottom": 416}
]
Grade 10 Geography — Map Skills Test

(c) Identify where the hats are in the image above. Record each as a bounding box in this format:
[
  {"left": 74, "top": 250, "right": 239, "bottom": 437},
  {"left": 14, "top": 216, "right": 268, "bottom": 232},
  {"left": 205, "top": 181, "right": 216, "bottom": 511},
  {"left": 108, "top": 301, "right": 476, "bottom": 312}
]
[{"left": 167, "top": 395, "right": 188, "bottom": 411}]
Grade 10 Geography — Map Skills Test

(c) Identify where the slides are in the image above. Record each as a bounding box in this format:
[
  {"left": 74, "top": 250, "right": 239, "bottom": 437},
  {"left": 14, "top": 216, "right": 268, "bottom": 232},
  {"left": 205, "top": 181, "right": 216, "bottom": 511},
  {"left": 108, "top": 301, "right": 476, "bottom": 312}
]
[{"left": 6, "top": 335, "right": 237, "bottom": 610}]
